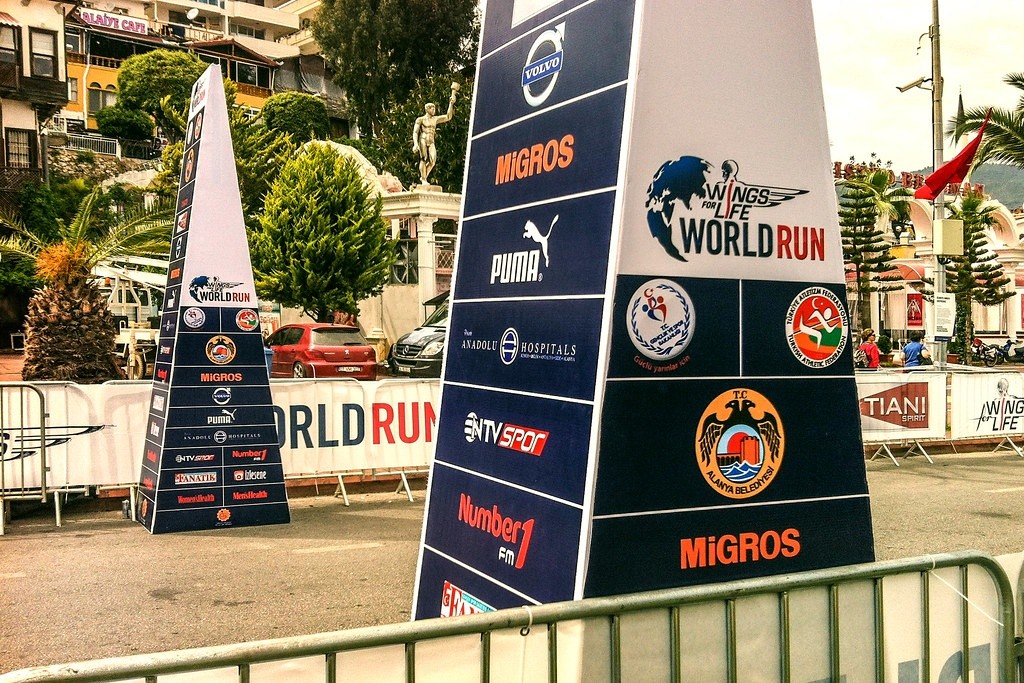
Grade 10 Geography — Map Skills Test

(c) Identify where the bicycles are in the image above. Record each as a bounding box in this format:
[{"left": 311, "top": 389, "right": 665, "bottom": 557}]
[{"left": 969, "top": 335, "right": 998, "bottom": 367}]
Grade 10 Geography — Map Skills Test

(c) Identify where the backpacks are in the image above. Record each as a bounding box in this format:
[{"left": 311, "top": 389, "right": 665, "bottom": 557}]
[{"left": 853, "top": 348, "right": 869, "bottom": 369}]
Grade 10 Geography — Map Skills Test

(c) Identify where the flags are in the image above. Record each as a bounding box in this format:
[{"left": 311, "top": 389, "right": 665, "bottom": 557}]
[{"left": 913, "top": 106, "right": 993, "bottom": 200}]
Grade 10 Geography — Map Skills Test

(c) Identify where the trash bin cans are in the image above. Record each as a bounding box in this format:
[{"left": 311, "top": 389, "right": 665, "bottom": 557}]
[{"left": 265, "top": 348, "right": 274, "bottom": 377}]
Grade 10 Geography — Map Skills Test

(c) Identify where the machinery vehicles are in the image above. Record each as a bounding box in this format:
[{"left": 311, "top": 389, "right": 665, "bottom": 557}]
[{"left": 78, "top": 252, "right": 169, "bottom": 380}]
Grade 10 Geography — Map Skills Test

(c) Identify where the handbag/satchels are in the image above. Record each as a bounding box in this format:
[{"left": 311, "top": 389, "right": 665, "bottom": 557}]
[{"left": 916, "top": 345, "right": 933, "bottom": 365}]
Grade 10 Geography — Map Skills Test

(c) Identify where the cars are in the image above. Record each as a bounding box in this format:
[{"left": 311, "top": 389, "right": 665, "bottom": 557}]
[
  {"left": 263, "top": 323, "right": 379, "bottom": 382},
  {"left": 386, "top": 296, "right": 449, "bottom": 379}
]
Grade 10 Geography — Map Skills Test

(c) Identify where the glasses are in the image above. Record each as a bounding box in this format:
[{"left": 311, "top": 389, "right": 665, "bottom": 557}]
[{"left": 871, "top": 334, "right": 875, "bottom": 337}]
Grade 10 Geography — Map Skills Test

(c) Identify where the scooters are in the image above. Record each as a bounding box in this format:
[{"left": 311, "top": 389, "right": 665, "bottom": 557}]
[{"left": 995, "top": 338, "right": 1024, "bottom": 366}]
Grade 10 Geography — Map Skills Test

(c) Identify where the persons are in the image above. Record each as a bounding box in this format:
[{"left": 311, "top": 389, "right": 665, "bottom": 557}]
[
  {"left": 900, "top": 331, "right": 929, "bottom": 367},
  {"left": 853, "top": 329, "right": 881, "bottom": 368}
]
